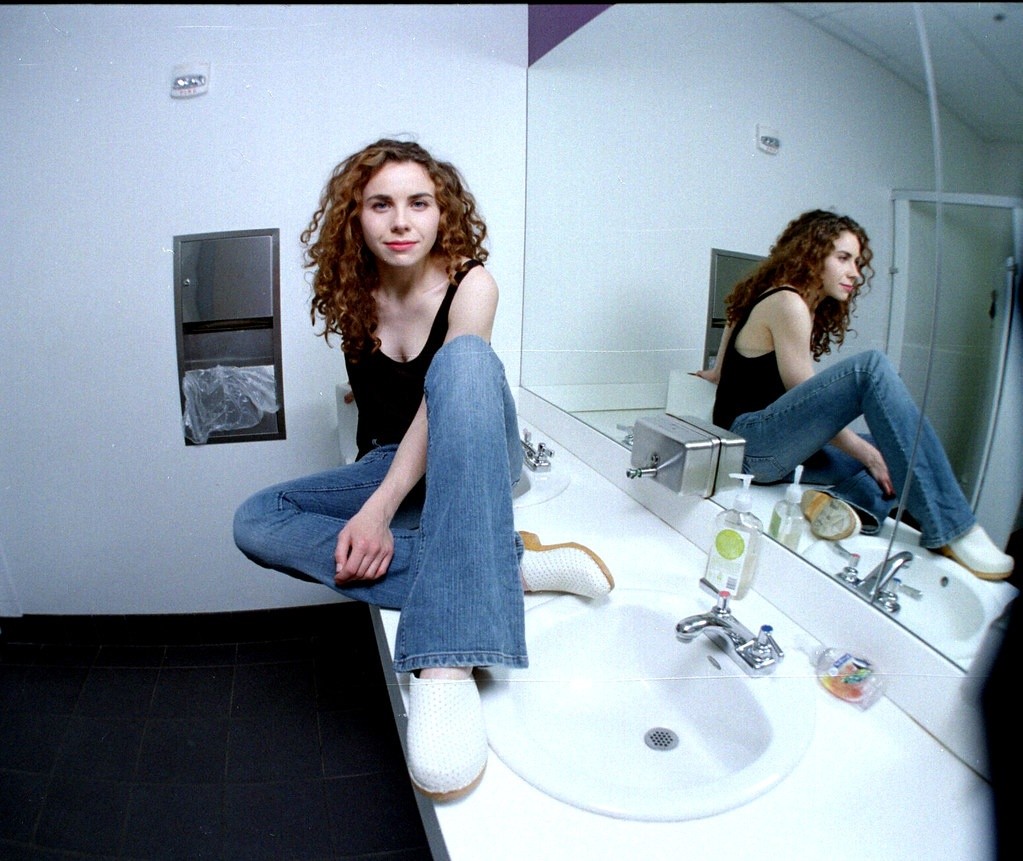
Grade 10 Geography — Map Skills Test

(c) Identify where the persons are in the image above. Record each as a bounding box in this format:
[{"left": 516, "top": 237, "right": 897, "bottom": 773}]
[
  {"left": 233, "top": 137, "right": 615, "bottom": 795},
  {"left": 685, "top": 206, "right": 1015, "bottom": 578}
]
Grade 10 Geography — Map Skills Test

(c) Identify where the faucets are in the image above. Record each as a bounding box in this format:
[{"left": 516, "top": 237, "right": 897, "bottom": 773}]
[
  {"left": 673, "top": 574, "right": 762, "bottom": 664},
  {"left": 517, "top": 440, "right": 542, "bottom": 463},
  {"left": 850, "top": 547, "right": 916, "bottom": 604}
]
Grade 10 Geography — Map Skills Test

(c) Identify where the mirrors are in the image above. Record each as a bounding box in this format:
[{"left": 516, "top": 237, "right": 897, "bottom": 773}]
[{"left": 518, "top": 0, "right": 1023, "bottom": 707}]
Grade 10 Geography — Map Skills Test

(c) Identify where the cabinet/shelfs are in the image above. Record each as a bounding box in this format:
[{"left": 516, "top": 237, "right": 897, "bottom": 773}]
[{"left": 172, "top": 226, "right": 287, "bottom": 446}]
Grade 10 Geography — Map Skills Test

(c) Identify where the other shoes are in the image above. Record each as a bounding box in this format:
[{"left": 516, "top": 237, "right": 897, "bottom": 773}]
[
  {"left": 801, "top": 490, "right": 864, "bottom": 541},
  {"left": 406, "top": 671, "right": 490, "bottom": 800},
  {"left": 942, "top": 524, "right": 1013, "bottom": 580},
  {"left": 513, "top": 531, "right": 615, "bottom": 598}
]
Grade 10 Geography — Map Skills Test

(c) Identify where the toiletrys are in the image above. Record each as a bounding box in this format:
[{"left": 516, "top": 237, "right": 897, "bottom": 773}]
[
  {"left": 703, "top": 471, "right": 764, "bottom": 607},
  {"left": 675, "top": 415, "right": 745, "bottom": 495},
  {"left": 791, "top": 633, "right": 887, "bottom": 708},
  {"left": 625, "top": 414, "right": 718, "bottom": 504},
  {"left": 771, "top": 462, "right": 811, "bottom": 558}
]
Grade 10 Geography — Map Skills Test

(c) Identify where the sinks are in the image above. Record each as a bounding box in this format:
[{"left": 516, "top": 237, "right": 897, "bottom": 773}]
[
  {"left": 457, "top": 583, "right": 820, "bottom": 832},
  {"left": 798, "top": 526, "right": 997, "bottom": 664},
  {"left": 502, "top": 438, "right": 588, "bottom": 523}
]
